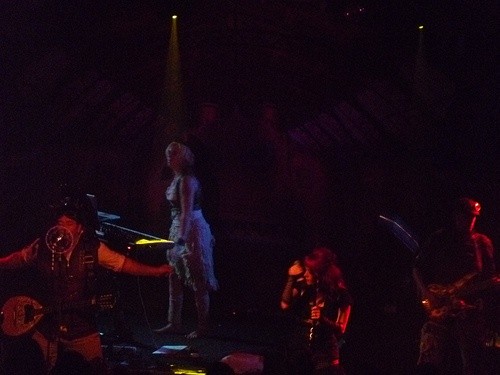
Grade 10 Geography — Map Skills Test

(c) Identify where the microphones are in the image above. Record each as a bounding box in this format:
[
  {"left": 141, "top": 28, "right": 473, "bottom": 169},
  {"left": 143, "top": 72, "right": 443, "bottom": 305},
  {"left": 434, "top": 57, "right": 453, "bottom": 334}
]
[{"left": 45, "top": 226, "right": 74, "bottom": 254}]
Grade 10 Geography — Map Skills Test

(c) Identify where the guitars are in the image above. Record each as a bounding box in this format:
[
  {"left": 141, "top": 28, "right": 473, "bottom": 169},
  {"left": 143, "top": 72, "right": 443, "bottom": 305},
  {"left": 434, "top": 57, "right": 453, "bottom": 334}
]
[
  {"left": 1, "top": 291, "right": 115, "bottom": 337},
  {"left": 416, "top": 268, "right": 475, "bottom": 323}
]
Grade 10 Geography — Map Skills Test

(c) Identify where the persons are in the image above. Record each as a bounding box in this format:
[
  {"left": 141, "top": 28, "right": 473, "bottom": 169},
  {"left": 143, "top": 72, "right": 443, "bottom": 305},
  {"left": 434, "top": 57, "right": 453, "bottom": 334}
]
[
  {"left": 0, "top": 187, "right": 175, "bottom": 375},
  {"left": 412, "top": 194, "right": 500, "bottom": 375},
  {"left": 150, "top": 142, "right": 219, "bottom": 341},
  {"left": 279, "top": 244, "right": 351, "bottom": 369}
]
